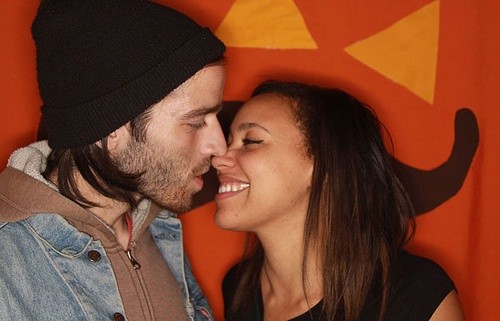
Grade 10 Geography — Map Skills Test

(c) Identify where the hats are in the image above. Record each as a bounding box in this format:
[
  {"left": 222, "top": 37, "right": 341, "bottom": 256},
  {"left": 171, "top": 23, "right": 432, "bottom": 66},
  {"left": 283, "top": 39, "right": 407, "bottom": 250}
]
[{"left": 30, "top": 0, "right": 227, "bottom": 150}]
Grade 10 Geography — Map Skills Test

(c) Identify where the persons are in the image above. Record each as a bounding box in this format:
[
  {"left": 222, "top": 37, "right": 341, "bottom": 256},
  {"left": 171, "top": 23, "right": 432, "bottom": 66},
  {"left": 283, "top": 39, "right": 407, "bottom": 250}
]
[
  {"left": 0, "top": 0, "right": 228, "bottom": 321},
  {"left": 210, "top": 79, "right": 465, "bottom": 321}
]
